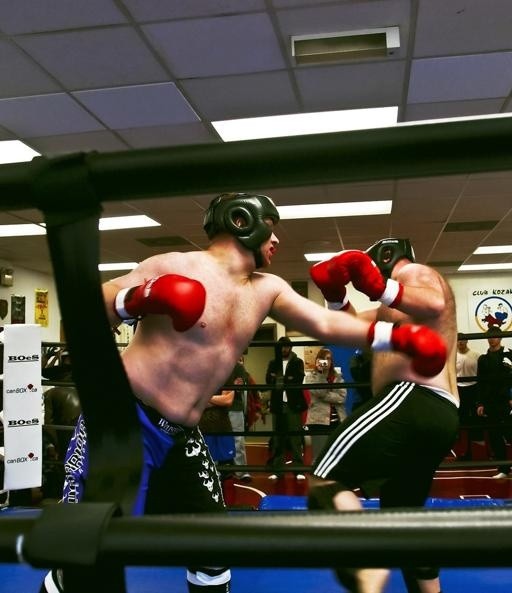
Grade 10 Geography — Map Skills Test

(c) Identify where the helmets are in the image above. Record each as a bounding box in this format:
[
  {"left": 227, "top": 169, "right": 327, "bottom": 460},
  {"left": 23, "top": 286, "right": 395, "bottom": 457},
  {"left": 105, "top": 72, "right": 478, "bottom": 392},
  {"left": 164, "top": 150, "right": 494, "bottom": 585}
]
[
  {"left": 365, "top": 238, "right": 417, "bottom": 277},
  {"left": 202, "top": 192, "right": 279, "bottom": 249}
]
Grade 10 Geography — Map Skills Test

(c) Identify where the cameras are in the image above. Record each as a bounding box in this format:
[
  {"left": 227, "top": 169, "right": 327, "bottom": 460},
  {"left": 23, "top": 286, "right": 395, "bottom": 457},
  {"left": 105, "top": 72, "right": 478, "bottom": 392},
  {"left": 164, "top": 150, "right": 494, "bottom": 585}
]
[{"left": 318, "top": 360, "right": 328, "bottom": 367}]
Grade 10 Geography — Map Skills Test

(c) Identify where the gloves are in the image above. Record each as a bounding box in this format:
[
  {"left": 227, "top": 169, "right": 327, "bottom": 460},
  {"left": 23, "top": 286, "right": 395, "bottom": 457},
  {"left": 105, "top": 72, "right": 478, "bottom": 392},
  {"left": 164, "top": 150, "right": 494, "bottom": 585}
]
[
  {"left": 366, "top": 320, "right": 447, "bottom": 378},
  {"left": 309, "top": 249, "right": 404, "bottom": 312},
  {"left": 113, "top": 273, "right": 206, "bottom": 332}
]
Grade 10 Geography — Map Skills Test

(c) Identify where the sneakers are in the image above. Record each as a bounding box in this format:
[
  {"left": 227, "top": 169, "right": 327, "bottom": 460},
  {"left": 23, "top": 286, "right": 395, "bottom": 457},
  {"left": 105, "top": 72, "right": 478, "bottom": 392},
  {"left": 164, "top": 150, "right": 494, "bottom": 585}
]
[
  {"left": 296, "top": 474, "right": 305, "bottom": 480},
  {"left": 492, "top": 473, "right": 507, "bottom": 479},
  {"left": 240, "top": 473, "right": 251, "bottom": 481},
  {"left": 268, "top": 475, "right": 278, "bottom": 480}
]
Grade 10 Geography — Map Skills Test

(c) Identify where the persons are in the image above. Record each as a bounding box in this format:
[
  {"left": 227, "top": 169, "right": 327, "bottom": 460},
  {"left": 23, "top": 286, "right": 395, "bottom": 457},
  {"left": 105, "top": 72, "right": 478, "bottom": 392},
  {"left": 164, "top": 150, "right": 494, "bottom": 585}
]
[
  {"left": 312, "top": 237, "right": 462, "bottom": 592},
  {"left": 475, "top": 327, "right": 512, "bottom": 478},
  {"left": 198, "top": 336, "right": 348, "bottom": 482},
  {"left": 38, "top": 193, "right": 448, "bottom": 592},
  {"left": 456, "top": 333, "right": 485, "bottom": 445}
]
